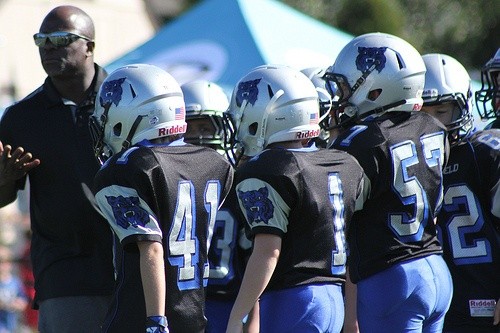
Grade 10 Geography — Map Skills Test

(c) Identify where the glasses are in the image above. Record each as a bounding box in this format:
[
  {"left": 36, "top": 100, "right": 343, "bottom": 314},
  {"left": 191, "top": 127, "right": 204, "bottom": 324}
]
[{"left": 33, "top": 32, "right": 90, "bottom": 46}]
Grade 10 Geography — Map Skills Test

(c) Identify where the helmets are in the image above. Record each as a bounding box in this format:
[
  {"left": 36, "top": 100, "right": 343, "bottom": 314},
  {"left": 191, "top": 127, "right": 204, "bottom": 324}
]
[
  {"left": 88, "top": 62, "right": 187, "bottom": 165},
  {"left": 180, "top": 79, "right": 231, "bottom": 144},
  {"left": 317, "top": 31, "right": 427, "bottom": 130},
  {"left": 421, "top": 52, "right": 474, "bottom": 148},
  {"left": 222, "top": 63, "right": 320, "bottom": 171},
  {"left": 299, "top": 66, "right": 340, "bottom": 125},
  {"left": 475, "top": 47, "right": 500, "bottom": 121}
]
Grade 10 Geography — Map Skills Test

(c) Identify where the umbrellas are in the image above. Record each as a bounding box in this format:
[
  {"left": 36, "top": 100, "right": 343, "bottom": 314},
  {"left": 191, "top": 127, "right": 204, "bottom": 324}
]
[{"left": 101, "top": 0, "right": 356, "bottom": 114}]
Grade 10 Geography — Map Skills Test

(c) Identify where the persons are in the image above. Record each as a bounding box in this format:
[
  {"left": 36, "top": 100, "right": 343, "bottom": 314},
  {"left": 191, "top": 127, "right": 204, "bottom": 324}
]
[
  {"left": 181, "top": 80, "right": 263, "bottom": 333},
  {"left": 420, "top": 53, "right": 500, "bottom": 333},
  {"left": 482, "top": 48, "right": 500, "bottom": 130},
  {"left": 323, "top": 32, "right": 453, "bottom": 333},
  {"left": 0, "top": 7, "right": 114, "bottom": 333},
  {"left": 225, "top": 65, "right": 350, "bottom": 333},
  {"left": 81, "top": 62, "right": 207, "bottom": 333}
]
